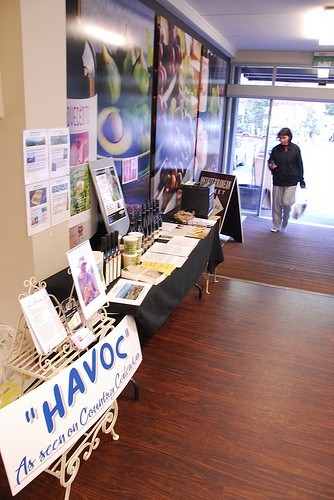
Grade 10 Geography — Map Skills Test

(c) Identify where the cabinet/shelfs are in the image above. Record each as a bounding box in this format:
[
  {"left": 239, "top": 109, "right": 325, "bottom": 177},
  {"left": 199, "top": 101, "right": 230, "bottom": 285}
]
[{"left": 0, "top": 266, "right": 121, "bottom": 500}]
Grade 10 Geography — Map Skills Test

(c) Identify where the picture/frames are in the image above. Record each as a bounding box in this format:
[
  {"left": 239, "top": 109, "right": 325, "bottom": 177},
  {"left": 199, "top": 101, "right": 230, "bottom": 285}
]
[{"left": 65, "top": 239, "right": 109, "bottom": 319}]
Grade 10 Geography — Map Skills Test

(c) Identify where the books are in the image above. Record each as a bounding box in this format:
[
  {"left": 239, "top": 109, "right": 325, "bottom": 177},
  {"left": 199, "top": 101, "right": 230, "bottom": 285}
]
[{"left": 107, "top": 218, "right": 217, "bottom": 306}]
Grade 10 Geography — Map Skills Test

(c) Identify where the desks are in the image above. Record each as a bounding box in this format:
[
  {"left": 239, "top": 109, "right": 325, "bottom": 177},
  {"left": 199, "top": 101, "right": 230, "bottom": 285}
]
[{"left": 32, "top": 212, "right": 225, "bottom": 401}]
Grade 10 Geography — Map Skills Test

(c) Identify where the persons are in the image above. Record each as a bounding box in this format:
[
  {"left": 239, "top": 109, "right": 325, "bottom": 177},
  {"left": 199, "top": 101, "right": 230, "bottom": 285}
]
[{"left": 268, "top": 127, "right": 306, "bottom": 233}]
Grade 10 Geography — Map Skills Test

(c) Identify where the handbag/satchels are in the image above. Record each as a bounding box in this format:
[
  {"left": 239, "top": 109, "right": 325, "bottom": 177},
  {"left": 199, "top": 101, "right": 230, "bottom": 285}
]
[{"left": 292, "top": 185, "right": 308, "bottom": 219}]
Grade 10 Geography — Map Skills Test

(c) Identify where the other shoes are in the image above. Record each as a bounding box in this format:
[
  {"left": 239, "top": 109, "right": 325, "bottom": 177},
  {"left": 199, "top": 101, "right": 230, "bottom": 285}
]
[
  {"left": 280, "top": 227, "right": 286, "bottom": 232},
  {"left": 271, "top": 227, "right": 278, "bottom": 232}
]
